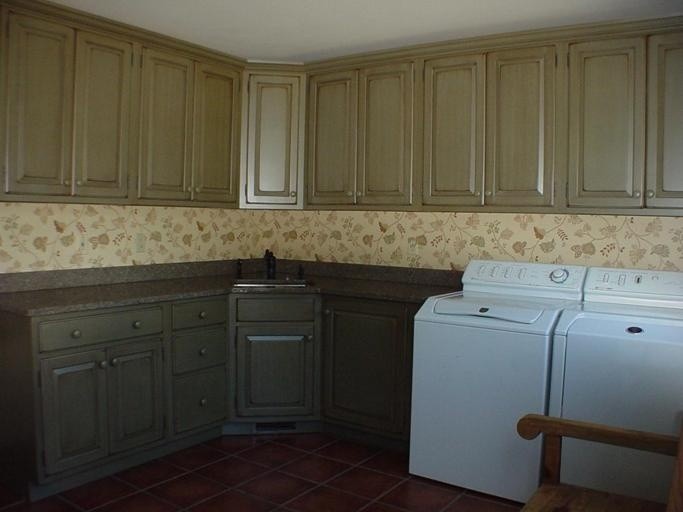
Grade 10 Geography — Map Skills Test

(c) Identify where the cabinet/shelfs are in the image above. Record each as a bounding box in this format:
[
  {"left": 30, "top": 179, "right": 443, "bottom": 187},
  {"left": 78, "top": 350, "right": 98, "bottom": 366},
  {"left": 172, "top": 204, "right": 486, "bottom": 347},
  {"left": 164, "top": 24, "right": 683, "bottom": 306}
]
[
  {"left": 311, "top": 273, "right": 463, "bottom": 446},
  {"left": 230, "top": 271, "right": 321, "bottom": 437},
  {"left": 555, "top": 16, "right": 683, "bottom": 217},
  {"left": 140, "top": 24, "right": 243, "bottom": 218},
  {"left": 414, "top": 28, "right": 555, "bottom": 213},
  {"left": 12, "top": 298, "right": 171, "bottom": 506},
  {"left": 241, "top": 58, "right": 305, "bottom": 211},
  {"left": 0, "top": 2, "right": 136, "bottom": 213},
  {"left": 162, "top": 273, "right": 226, "bottom": 449},
  {"left": 307, "top": 47, "right": 414, "bottom": 210}
]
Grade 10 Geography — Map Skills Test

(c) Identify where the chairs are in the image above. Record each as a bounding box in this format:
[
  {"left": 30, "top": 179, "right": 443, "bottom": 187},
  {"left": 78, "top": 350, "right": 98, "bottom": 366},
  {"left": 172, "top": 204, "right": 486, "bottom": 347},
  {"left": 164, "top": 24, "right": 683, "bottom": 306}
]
[{"left": 515, "top": 416, "right": 683, "bottom": 512}]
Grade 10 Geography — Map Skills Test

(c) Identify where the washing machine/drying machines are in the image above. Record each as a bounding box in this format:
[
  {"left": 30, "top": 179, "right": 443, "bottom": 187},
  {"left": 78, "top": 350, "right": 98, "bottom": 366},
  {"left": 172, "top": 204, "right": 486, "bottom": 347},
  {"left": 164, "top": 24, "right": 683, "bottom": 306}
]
[
  {"left": 408, "top": 259, "right": 586, "bottom": 504},
  {"left": 548, "top": 267, "right": 683, "bottom": 506}
]
